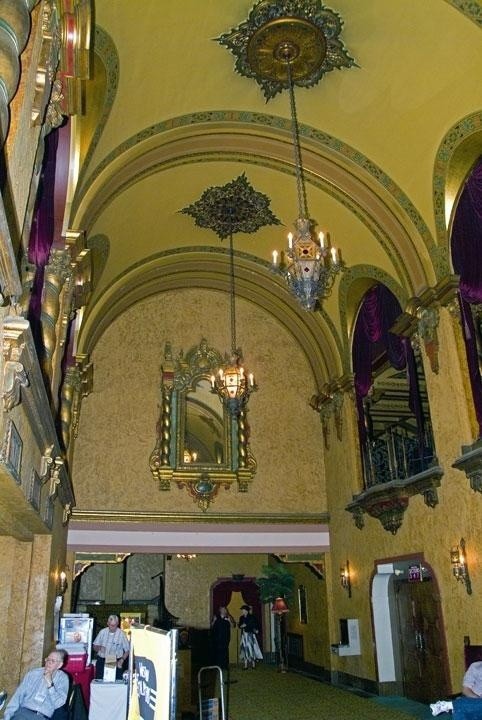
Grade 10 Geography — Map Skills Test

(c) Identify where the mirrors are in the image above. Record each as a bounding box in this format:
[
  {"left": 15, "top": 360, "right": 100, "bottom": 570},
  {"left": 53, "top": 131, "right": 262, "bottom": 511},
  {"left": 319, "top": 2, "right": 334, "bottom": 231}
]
[{"left": 149, "top": 336, "right": 258, "bottom": 512}]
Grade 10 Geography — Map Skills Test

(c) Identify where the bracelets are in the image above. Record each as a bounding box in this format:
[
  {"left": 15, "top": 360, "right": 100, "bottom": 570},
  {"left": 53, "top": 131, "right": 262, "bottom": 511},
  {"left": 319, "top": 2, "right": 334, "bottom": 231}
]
[
  {"left": 120, "top": 657, "right": 125, "bottom": 663},
  {"left": 48, "top": 683, "right": 55, "bottom": 690}
]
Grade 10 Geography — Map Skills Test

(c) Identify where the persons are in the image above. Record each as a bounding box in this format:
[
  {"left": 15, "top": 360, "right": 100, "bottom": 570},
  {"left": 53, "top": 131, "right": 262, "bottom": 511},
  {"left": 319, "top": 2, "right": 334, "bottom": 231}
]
[
  {"left": 463, "top": 660, "right": 482, "bottom": 699},
  {"left": 3, "top": 650, "right": 69, "bottom": 720},
  {"left": 93, "top": 614, "right": 130, "bottom": 681},
  {"left": 209, "top": 604, "right": 263, "bottom": 671}
]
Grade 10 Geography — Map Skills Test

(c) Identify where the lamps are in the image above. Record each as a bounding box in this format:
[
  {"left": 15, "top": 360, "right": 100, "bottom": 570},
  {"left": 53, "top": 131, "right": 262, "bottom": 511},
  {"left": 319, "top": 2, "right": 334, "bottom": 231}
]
[
  {"left": 210, "top": 266, "right": 257, "bottom": 421},
  {"left": 340, "top": 560, "right": 352, "bottom": 599},
  {"left": 272, "top": 597, "right": 289, "bottom": 674},
  {"left": 450, "top": 536, "right": 473, "bottom": 596},
  {"left": 267, "top": 46, "right": 350, "bottom": 315}
]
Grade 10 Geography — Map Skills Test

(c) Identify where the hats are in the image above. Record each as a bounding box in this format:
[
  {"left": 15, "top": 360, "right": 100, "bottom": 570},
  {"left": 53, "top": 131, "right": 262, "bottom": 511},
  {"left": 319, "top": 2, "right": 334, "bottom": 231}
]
[{"left": 239, "top": 605, "right": 250, "bottom": 612}]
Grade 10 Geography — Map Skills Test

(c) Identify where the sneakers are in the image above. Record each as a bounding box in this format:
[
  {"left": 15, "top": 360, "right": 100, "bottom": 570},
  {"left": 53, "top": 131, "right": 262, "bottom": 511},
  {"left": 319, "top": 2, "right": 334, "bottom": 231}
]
[{"left": 429, "top": 700, "right": 450, "bottom": 717}]
